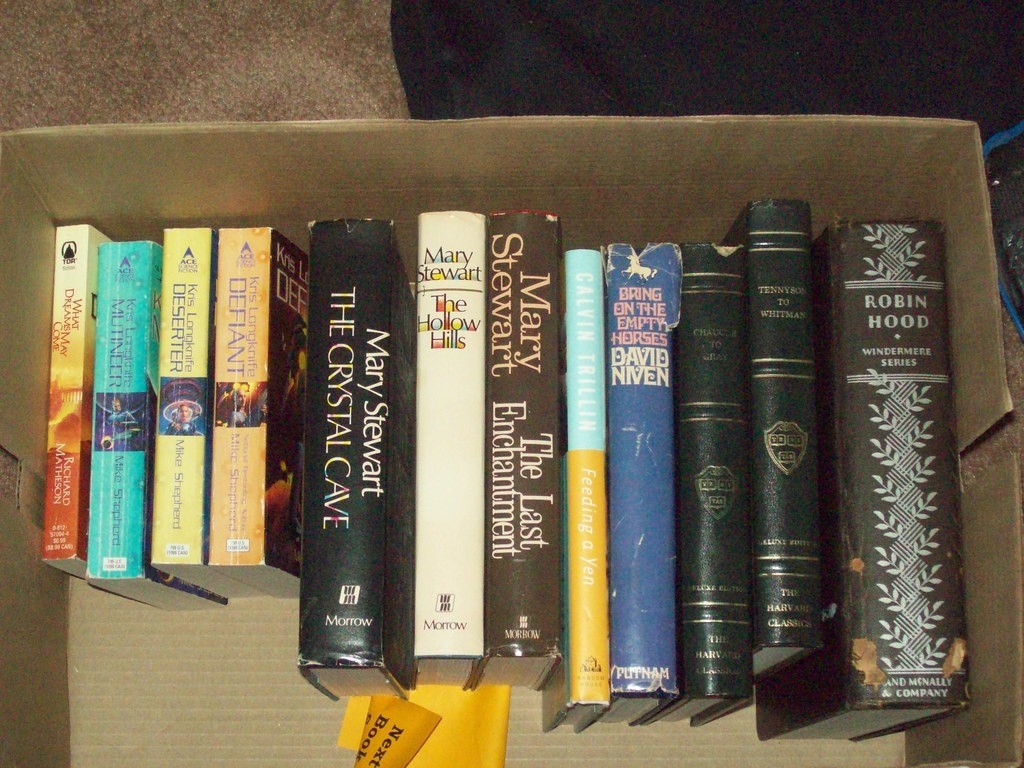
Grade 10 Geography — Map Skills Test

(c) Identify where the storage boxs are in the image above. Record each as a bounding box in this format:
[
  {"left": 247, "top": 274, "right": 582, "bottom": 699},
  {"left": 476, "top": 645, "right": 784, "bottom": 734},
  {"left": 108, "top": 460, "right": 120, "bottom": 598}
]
[{"left": 0, "top": 115, "right": 1024, "bottom": 768}]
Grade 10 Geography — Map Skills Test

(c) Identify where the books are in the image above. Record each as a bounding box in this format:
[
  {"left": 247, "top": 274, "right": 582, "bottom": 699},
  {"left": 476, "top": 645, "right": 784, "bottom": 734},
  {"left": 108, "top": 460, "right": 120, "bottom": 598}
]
[
  {"left": 756, "top": 219, "right": 971, "bottom": 742},
  {"left": 746, "top": 198, "right": 827, "bottom": 677},
  {"left": 601, "top": 239, "right": 687, "bottom": 728},
  {"left": 151, "top": 228, "right": 308, "bottom": 600},
  {"left": 41, "top": 225, "right": 114, "bottom": 578},
  {"left": 297, "top": 217, "right": 416, "bottom": 701},
  {"left": 473, "top": 211, "right": 560, "bottom": 696},
  {"left": 539, "top": 248, "right": 609, "bottom": 734},
  {"left": 643, "top": 244, "right": 751, "bottom": 728},
  {"left": 415, "top": 211, "right": 487, "bottom": 693},
  {"left": 84, "top": 240, "right": 229, "bottom": 609}
]
